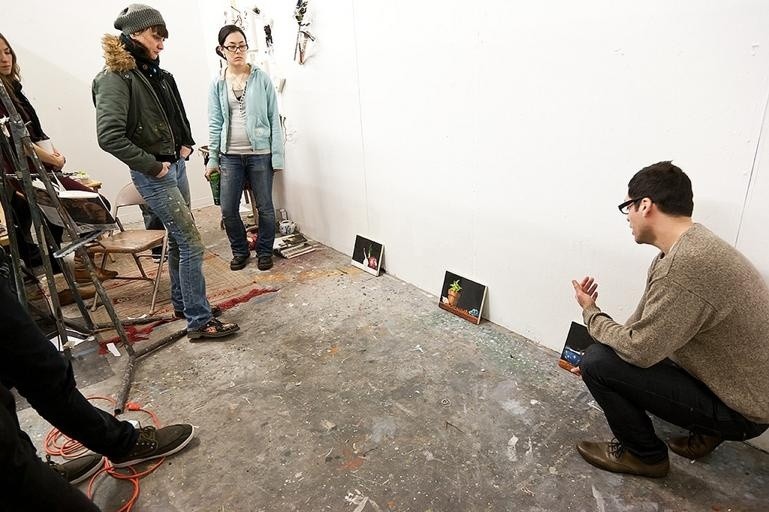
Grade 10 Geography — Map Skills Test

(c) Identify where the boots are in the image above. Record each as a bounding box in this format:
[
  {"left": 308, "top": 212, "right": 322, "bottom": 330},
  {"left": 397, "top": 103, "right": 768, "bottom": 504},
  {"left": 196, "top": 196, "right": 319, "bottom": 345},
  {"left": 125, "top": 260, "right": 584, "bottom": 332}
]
[
  {"left": 41, "top": 273, "right": 96, "bottom": 306},
  {"left": 74, "top": 251, "right": 118, "bottom": 283}
]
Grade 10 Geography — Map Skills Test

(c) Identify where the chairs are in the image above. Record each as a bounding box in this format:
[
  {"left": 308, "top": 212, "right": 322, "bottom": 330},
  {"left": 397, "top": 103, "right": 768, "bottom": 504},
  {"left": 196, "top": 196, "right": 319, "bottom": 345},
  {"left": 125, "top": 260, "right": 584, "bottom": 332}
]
[{"left": 5, "top": 173, "right": 173, "bottom": 316}]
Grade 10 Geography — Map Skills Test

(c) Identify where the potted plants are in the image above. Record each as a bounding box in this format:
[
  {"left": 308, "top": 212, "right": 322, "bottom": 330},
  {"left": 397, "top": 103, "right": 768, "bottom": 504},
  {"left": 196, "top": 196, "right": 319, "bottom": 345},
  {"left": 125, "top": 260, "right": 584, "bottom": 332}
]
[{"left": 448, "top": 279, "right": 464, "bottom": 307}]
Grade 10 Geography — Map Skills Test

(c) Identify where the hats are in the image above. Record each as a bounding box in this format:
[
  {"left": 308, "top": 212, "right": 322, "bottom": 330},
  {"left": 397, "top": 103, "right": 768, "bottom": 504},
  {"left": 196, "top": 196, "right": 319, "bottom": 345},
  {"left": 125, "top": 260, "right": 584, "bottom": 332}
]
[{"left": 114, "top": 3, "right": 165, "bottom": 35}]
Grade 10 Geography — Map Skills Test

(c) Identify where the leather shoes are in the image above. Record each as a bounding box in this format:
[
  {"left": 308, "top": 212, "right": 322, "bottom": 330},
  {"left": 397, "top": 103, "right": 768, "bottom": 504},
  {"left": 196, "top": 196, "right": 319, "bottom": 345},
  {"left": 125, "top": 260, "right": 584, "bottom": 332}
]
[
  {"left": 173, "top": 305, "right": 221, "bottom": 317},
  {"left": 668, "top": 434, "right": 725, "bottom": 460},
  {"left": 188, "top": 318, "right": 240, "bottom": 339},
  {"left": 576, "top": 441, "right": 669, "bottom": 478}
]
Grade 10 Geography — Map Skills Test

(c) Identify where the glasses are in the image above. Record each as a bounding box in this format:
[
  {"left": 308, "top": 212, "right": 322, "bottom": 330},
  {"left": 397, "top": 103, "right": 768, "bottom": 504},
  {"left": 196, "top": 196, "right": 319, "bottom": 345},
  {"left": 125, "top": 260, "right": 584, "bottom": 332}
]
[
  {"left": 618, "top": 196, "right": 654, "bottom": 214},
  {"left": 223, "top": 44, "right": 248, "bottom": 51}
]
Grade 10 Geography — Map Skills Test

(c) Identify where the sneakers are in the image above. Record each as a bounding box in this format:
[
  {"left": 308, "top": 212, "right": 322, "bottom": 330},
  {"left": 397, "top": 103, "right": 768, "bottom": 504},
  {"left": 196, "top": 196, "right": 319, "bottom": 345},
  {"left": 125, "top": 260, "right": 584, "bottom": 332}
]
[
  {"left": 258, "top": 256, "right": 272, "bottom": 270},
  {"left": 231, "top": 253, "right": 250, "bottom": 270},
  {"left": 44, "top": 454, "right": 104, "bottom": 486},
  {"left": 108, "top": 424, "right": 194, "bottom": 469}
]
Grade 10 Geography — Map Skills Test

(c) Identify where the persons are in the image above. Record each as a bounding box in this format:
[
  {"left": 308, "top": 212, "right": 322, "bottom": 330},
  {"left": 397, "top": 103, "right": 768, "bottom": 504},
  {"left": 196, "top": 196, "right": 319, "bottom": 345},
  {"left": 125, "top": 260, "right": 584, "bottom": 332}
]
[
  {"left": 203, "top": 25, "right": 285, "bottom": 270},
  {"left": 92, "top": 4, "right": 240, "bottom": 340},
  {"left": 0, "top": 32, "right": 118, "bottom": 286},
  {"left": 571, "top": 160, "right": 769, "bottom": 478},
  {"left": 0, "top": 281, "right": 195, "bottom": 512}
]
